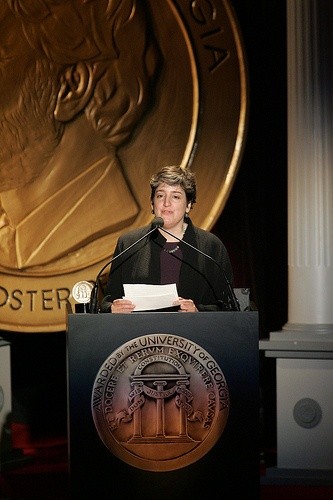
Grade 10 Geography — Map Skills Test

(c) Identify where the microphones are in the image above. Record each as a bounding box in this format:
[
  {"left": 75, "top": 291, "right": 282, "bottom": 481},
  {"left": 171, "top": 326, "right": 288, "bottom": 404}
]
[{"left": 88, "top": 218, "right": 240, "bottom": 314}]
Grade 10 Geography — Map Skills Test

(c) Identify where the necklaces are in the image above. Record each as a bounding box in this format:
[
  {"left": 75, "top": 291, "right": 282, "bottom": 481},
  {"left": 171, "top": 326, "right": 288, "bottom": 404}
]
[{"left": 162, "top": 225, "right": 186, "bottom": 254}]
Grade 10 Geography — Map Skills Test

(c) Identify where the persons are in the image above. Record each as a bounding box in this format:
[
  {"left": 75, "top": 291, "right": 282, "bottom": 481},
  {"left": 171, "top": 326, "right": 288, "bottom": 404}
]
[{"left": 97, "top": 166, "right": 243, "bottom": 314}]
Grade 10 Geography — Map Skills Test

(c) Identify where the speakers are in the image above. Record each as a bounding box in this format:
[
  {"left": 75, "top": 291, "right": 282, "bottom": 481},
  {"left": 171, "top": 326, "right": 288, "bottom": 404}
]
[{"left": 26, "top": 401, "right": 65, "bottom": 439}]
